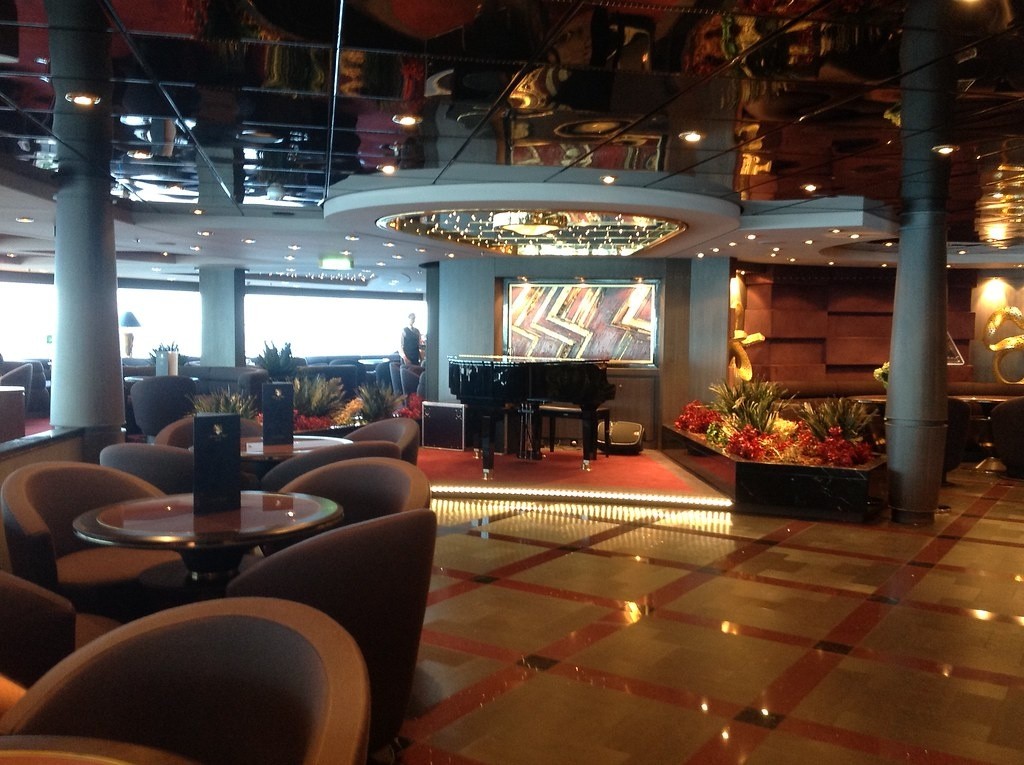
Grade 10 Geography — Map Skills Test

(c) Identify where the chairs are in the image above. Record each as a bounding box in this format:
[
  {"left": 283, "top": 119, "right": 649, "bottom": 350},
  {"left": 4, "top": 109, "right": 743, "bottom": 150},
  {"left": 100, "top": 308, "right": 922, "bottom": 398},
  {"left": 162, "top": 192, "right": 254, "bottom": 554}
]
[
  {"left": 990, "top": 396, "right": 1024, "bottom": 481},
  {"left": 1, "top": 359, "right": 437, "bottom": 765},
  {"left": 941, "top": 397, "right": 971, "bottom": 486}
]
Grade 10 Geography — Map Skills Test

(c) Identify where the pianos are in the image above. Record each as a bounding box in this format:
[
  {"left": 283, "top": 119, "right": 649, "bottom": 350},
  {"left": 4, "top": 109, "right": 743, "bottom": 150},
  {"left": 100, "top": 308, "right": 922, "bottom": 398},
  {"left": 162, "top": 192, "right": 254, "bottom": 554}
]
[{"left": 448, "top": 359, "right": 617, "bottom": 480}]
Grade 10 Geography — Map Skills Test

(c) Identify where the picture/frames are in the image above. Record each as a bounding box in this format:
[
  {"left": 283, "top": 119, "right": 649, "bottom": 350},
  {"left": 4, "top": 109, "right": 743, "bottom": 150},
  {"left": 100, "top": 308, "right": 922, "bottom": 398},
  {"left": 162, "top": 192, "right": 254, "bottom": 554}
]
[{"left": 502, "top": 278, "right": 661, "bottom": 369}]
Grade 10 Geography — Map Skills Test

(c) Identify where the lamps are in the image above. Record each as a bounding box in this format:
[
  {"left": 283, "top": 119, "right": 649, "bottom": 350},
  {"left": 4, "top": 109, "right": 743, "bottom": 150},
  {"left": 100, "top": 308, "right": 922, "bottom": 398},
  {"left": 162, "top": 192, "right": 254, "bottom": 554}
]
[
  {"left": 800, "top": 183, "right": 821, "bottom": 192},
  {"left": 16, "top": 217, "right": 34, "bottom": 223},
  {"left": 190, "top": 208, "right": 208, "bottom": 216},
  {"left": 678, "top": 131, "right": 708, "bottom": 143},
  {"left": 65, "top": 93, "right": 101, "bottom": 105},
  {"left": 119, "top": 312, "right": 141, "bottom": 359},
  {"left": 492, "top": 211, "right": 569, "bottom": 235},
  {"left": 376, "top": 163, "right": 398, "bottom": 176},
  {"left": 600, "top": 175, "right": 618, "bottom": 185},
  {"left": 391, "top": 114, "right": 423, "bottom": 126},
  {"left": 932, "top": 145, "right": 960, "bottom": 156},
  {"left": 197, "top": 232, "right": 214, "bottom": 237}
]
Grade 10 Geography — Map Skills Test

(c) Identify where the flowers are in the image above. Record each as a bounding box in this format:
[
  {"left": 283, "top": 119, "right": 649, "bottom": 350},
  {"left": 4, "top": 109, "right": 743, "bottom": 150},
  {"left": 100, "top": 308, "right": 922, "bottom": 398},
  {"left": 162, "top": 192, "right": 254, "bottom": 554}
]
[
  {"left": 874, "top": 362, "right": 890, "bottom": 389},
  {"left": 179, "top": 373, "right": 424, "bottom": 431},
  {"left": 674, "top": 377, "right": 881, "bottom": 467}
]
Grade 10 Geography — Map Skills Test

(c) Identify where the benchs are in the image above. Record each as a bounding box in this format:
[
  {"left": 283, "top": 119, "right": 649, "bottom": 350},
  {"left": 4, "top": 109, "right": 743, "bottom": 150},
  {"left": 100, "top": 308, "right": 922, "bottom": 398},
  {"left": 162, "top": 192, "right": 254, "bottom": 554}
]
[
  {"left": 744, "top": 381, "right": 1024, "bottom": 463},
  {"left": 123, "top": 365, "right": 269, "bottom": 415},
  {"left": 292, "top": 357, "right": 358, "bottom": 399},
  {"left": 529, "top": 405, "right": 611, "bottom": 461}
]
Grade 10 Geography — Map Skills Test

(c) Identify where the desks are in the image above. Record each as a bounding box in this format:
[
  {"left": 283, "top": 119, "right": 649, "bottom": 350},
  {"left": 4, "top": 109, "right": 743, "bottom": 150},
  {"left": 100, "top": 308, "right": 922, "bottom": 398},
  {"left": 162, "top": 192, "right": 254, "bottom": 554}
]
[
  {"left": 187, "top": 436, "right": 357, "bottom": 482},
  {"left": 71, "top": 489, "right": 344, "bottom": 608},
  {"left": 845, "top": 395, "right": 1024, "bottom": 471}
]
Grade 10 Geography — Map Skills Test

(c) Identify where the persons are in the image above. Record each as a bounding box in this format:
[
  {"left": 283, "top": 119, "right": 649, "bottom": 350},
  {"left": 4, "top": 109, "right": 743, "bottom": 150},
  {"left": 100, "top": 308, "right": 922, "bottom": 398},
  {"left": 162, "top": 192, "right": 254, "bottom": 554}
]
[{"left": 399, "top": 314, "right": 425, "bottom": 366}]
